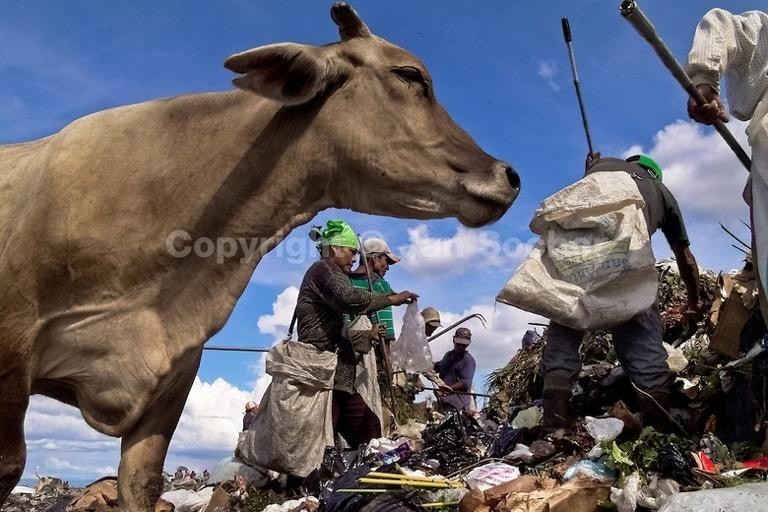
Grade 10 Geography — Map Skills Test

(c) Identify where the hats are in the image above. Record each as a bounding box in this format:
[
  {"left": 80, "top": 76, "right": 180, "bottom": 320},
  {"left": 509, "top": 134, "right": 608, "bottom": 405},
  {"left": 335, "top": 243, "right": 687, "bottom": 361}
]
[
  {"left": 454, "top": 328, "right": 471, "bottom": 345},
  {"left": 358, "top": 239, "right": 401, "bottom": 265},
  {"left": 625, "top": 155, "right": 662, "bottom": 181},
  {"left": 420, "top": 307, "right": 443, "bottom": 328},
  {"left": 245, "top": 402, "right": 259, "bottom": 411}
]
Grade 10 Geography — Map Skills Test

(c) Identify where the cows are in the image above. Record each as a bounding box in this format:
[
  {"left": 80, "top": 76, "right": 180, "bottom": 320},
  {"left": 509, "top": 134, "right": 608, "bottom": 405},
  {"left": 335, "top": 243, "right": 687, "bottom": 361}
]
[{"left": 1, "top": 0, "right": 523, "bottom": 512}]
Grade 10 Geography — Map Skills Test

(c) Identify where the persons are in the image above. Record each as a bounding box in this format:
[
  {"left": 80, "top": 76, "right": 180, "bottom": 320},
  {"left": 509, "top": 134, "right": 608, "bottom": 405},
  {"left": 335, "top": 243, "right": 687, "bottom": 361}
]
[
  {"left": 686, "top": 6, "right": 766, "bottom": 347},
  {"left": 344, "top": 237, "right": 396, "bottom": 382},
  {"left": 428, "top": 328, "right": 477, "bottom": 416},
  {"left": 389, "top": 306, "right": 454, "bottom": 398},
  {"left": 539, "top": 148, "right": 704, "bottom": 446},
  {"left": 296, "top": 219, "right": 420, "bottom": 450}
]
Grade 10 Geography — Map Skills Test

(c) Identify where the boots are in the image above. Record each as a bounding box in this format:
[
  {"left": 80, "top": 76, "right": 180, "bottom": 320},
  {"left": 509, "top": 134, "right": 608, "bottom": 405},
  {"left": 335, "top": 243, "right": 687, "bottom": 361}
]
[{"left": 542, "top": 378, "right": 573, "bottom": 432}]
